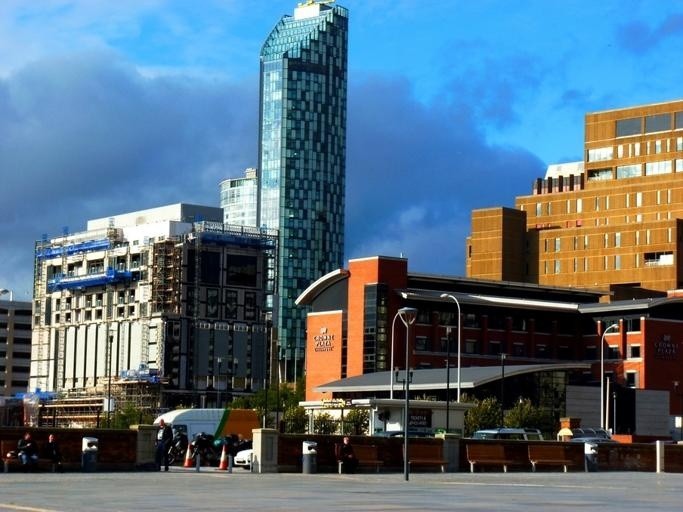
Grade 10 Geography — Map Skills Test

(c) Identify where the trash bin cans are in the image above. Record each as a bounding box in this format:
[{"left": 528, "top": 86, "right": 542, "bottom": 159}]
[
  {"left": 302, "top": 441, "right": 318, "bottom": 473},
  {"left": 81, "top": 436, "right": 99, "bottom": 472},
  {"left": 584, "top": 442, "right": 598, "bottom": 472}
]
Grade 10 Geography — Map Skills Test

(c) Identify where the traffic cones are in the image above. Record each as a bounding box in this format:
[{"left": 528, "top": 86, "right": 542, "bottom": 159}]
[
  {"left": 216, "top": 445, "right": 228, "bottom": 470},
  {"left": 183, "top": 442, "right": 193, "bottom": 468}
]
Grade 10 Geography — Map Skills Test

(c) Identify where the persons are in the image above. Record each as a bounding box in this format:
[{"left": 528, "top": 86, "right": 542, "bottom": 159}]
[
  {"left": 155, "top": 418, "right": 173, "bottom": 472},
  {"left": 16, "top": 432, "right": 45, "bottom": 467},
  {"left": 335, "top": 437, "right": 360, "bottom": 473},
  {"left": 42, "top": 434, "right": 62, "bottom": 472}
]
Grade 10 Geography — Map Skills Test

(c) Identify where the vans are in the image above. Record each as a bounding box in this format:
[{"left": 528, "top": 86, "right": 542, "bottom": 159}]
[
  {"left": 153, "top": 409, "right": 259, "bottom": 465},
  {"left": 473, "top": 428, "right": 544, "bottom": 440}
]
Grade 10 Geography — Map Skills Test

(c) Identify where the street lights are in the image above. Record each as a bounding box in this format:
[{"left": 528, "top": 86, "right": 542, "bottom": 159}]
[
  {"left": 395, "top": 307, "right": 418, "bottom": 480},
  {"left": 106, "top": 329, "right": 116, "bottom": 428},
  {"left": 499, "top": 352, "right": 512, "bottom": 427},
  {"left": 217, "top": 356, "right": 222, "bottom": 407},
  {"left": 601, "top": 324, "right": 620, "bottom": 429},
  {"left": 440, "top": 293, "right": 463, "bottom": 432}
]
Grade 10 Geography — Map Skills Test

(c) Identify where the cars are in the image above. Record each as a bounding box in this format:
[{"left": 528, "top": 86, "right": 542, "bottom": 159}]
[{"left": 234, "top": 449, "right": 257, "bottom": 470}]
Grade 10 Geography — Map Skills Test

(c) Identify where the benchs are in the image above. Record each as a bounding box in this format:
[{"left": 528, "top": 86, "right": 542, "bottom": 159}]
[
  {"left": 0, "top": 440, "right": 64, "bottom": 472},
  {"left": 334, "top": 442, "right": 573, "bottom": 473}
]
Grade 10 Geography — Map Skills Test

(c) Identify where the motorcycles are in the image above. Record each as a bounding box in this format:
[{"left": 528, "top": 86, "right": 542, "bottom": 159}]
[{"left": 168, "top": 428, "right": 252, "bottom": 466}]
